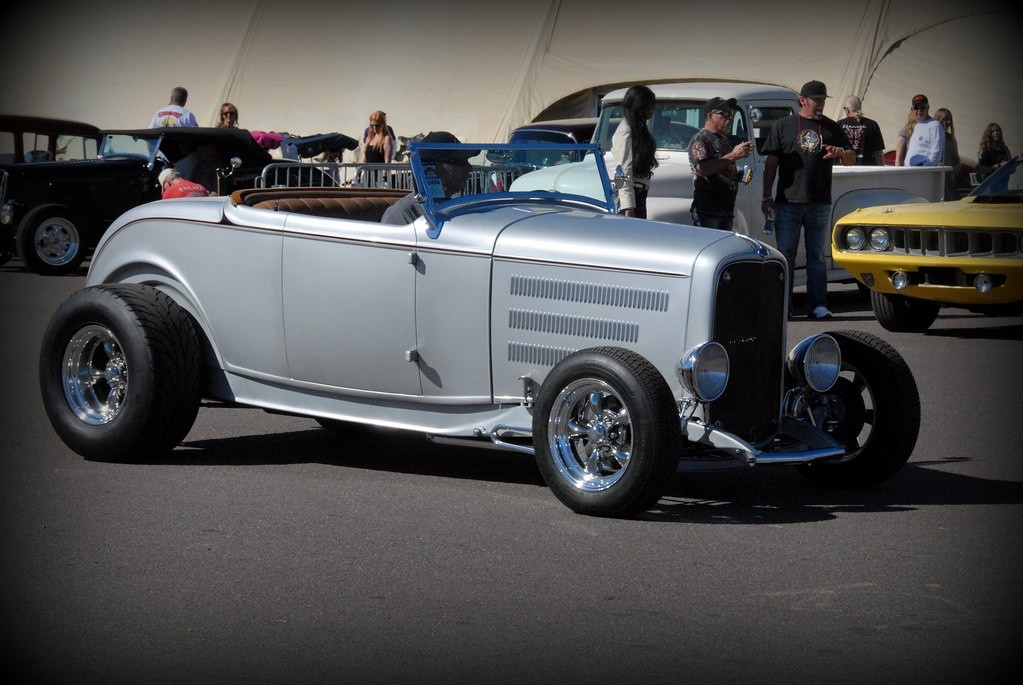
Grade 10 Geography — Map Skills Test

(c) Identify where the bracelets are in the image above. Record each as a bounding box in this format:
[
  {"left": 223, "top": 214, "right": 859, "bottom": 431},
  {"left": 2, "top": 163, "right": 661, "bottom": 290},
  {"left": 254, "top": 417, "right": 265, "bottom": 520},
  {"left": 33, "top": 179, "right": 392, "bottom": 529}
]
[{"left": 998, "top": 163, "right": 1001, "bottom": 169}]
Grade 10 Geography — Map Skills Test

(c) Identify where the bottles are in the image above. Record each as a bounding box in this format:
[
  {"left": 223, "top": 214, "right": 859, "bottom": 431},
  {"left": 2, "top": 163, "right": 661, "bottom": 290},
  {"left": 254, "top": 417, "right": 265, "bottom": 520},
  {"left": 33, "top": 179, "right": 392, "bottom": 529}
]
[{"left": 763, "top": 204, "right": 778, "bottom": 235}]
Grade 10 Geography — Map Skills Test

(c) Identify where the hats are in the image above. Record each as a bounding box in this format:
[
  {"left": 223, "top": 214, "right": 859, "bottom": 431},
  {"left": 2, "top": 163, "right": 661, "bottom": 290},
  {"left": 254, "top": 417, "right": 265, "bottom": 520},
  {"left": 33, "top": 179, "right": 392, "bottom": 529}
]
[
  {"left": 419, "top": 132, "right": 481, "bottom": 157},
  {"left": 800, "top": 81, "right": 831, "bottom": 98},
  {"left": 912, "top": 95, "right": 928, "bottom": 106},
  {"left": 704, "top": 97, "right": 737, "bottom": 112},
  {"left": 159, "top": 168, "right": 180, "bottom": 193}
]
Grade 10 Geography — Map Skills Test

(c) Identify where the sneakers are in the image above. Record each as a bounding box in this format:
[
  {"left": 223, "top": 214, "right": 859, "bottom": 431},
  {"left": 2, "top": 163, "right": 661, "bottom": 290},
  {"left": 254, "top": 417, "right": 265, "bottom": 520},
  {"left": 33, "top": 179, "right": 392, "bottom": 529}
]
[{"left": 808, "top": 305, "right": 832, "bottom": 319}]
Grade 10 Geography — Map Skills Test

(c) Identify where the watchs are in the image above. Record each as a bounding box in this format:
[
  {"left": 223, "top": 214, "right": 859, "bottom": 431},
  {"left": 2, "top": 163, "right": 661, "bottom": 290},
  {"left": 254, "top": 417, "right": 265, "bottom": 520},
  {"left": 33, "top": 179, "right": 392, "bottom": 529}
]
[{"left": 762, "top": 197, "right": 773, "bottom": 202}]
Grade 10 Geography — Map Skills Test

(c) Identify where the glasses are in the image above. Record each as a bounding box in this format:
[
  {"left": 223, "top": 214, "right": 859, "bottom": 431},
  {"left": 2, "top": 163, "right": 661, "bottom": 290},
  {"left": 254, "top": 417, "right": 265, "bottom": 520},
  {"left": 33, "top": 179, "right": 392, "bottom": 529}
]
[
  {"left": 914, "top": 105, "right": 926, "bottom": 109},
  {"left": 801, "top": 134, "right": 823, "bottom": 154},
  {"left": 436, "top": 157, "right": 467, "bottom": 166},
  {"left": 222, "top": 112, "right": 234, "bottom": 116},
  {"left": 712, "top": 111, "right": 732, "bottom": 117},
  {"left": 370, "top": 124, "right": 380, "bottom": 127}
]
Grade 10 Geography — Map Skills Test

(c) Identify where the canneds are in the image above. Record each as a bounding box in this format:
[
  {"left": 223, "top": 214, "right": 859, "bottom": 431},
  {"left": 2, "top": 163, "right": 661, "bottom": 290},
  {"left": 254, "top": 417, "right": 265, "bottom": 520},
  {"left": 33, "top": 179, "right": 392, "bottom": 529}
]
[{"left": 740, "top": 165, "right": 752, "bottom": 183}]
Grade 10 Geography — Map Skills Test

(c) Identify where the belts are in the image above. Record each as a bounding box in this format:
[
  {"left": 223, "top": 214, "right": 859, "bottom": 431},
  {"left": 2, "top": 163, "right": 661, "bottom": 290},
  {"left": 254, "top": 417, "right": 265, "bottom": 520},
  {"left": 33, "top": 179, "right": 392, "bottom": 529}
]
[{"left": 634, "top": 183, "right": 648, "bottom": 190}]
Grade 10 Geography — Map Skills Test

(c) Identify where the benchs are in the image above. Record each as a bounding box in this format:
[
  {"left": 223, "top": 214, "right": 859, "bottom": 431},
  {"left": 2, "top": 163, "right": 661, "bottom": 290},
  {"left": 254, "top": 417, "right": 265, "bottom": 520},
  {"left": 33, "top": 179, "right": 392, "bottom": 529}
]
[{"left": 246, "top": 191, "right": 408, "bottom": 224}]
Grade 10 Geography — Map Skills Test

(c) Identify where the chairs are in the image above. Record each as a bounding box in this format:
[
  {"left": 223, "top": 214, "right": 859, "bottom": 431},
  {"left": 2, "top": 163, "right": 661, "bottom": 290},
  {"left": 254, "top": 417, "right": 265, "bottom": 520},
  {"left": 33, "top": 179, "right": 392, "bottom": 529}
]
[
  {"left": 25, "top": 150, "right": 52, "bottom": 163},
  {"left": 0, "top": 153, "right": 15, "bottom": 164}
]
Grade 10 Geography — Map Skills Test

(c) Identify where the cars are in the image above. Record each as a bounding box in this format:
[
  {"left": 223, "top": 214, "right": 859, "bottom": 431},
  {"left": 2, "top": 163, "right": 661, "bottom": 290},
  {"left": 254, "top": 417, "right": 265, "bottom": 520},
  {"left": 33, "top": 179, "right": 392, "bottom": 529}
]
[
  {"left": 37, "top": 142, "right": 926, "bottom": 520},
  {"left": 0, "top": 113, "right": 361, "bottom": 276},
  {"left": 829, "top": 154, "right": 1023, "bottom": 334},
  {"left": 483, "top": 78, "right": 976, "bottom": 287}
]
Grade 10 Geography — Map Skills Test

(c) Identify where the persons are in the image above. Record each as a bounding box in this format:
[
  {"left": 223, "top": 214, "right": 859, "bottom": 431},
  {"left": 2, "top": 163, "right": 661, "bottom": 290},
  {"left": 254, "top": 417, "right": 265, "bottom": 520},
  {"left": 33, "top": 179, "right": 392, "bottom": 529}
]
[
  {"left": 364, "top": 112, "right": 397, "bottom": 160},
  {"left": 216, "top": 102, "right": 239, "bottom": 129},
  {"left": 381, "top": 131, "right": 473, "bottom": 225},
  {"left": 975, "top": 122, "right": 1012, "bottom": 192},
  {"left": 158, "top": 168, "right": 209, "bottom": 200},
  {"left": 147, "top": 87, "right": 198, "bottom": 163},
  {"left": 346, "top": 111, "right": 393, "bottom": 187},
  {"left": 761, "top": 80, "right": 856, "bottom": 319},
  {"left": 687, "top": 96, "right": 756, "bottom": 231},
  {"left": 836, "top": 95, "right": 885, "bottom": 166},
  {"left": 934, "top": 108, "right": 961, "bottom": 202},
  {"left": 611, "top": 85, "right": 659, "bottom": 219},
  {"left": 895, "top": 94, "right": 945, "bottom": 166}
]
